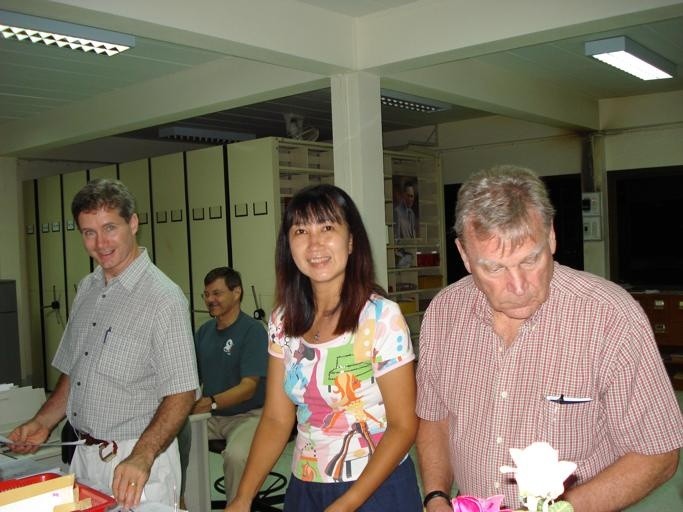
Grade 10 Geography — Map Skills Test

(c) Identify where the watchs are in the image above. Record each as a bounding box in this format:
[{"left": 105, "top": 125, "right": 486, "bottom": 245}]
[{"left": 210, "top": 396, "right": 217, "bottom": 411}]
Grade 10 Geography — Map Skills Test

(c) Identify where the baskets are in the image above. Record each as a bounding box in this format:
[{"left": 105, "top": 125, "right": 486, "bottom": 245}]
[{"left": 0, "top": 472, "right": 117, "bottom": 512}]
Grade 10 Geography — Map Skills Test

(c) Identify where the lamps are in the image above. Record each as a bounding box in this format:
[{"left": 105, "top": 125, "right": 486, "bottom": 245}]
[{"left": 583, "top": 34, "right": 677, "bottom": 82}]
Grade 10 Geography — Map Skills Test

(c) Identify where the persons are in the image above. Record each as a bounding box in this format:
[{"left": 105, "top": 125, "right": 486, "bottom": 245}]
[
  {"left": 8, "top": 176, "right": 198, "bottom": 512},
  {"left": 223, "top": 184, "right": 424, "bottom": 512},
  {"left": 191, "top": 266, "right": 268, "bottom": 508},
  {"left": 394, "top": 181, "right": 417, "bottom": 243},
  {"left": 416, "top": 164, "right": 683, "bottom": 512}
]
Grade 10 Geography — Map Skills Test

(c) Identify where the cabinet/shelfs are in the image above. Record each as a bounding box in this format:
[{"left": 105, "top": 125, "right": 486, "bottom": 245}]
[{"left": 19, "top": 135, "right": 449, "bottom": 393}]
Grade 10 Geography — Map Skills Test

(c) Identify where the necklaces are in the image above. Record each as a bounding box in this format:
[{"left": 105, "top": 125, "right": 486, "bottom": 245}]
[{"left": 302, "top": 306, "right": 338, "bottom": 341}]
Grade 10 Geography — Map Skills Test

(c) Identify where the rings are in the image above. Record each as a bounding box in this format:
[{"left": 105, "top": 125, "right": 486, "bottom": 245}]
[{"left": 130, "top": 483, "right": 136, "bottom": 487}]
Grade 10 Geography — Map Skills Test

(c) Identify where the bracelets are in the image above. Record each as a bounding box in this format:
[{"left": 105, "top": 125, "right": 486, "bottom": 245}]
[{"left": 424, "top": 491, "right": 450, "bottom": 507}]
